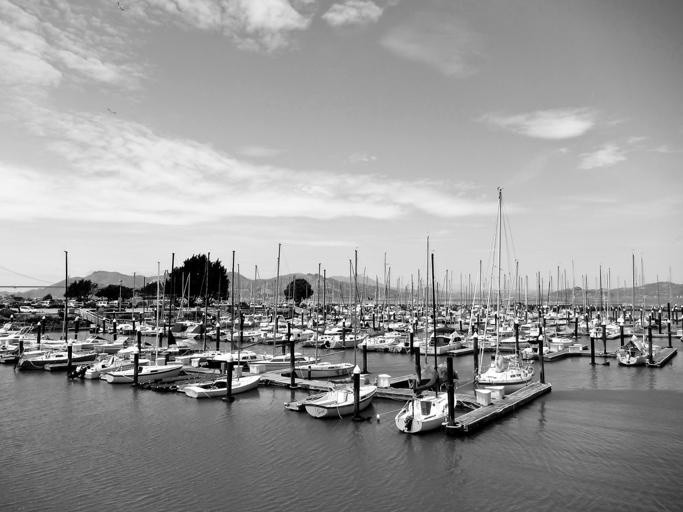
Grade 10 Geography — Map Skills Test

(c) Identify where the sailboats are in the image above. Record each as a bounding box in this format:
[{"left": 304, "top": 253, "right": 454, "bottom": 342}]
[
  {"left": 0, "top": 234, "right": 672, "bottom": 391},
  {"left": 470, "top": 187, "right": 536, "bottom": 396},
  {"left": 387, "top": 252, "right": 458, "bottom": 436},
  {"left": 186, "top": 250, "right": 263, "bottom": 400},
  {"left": 298, "top": 250, "right": 379, "bottom": 421}
]
